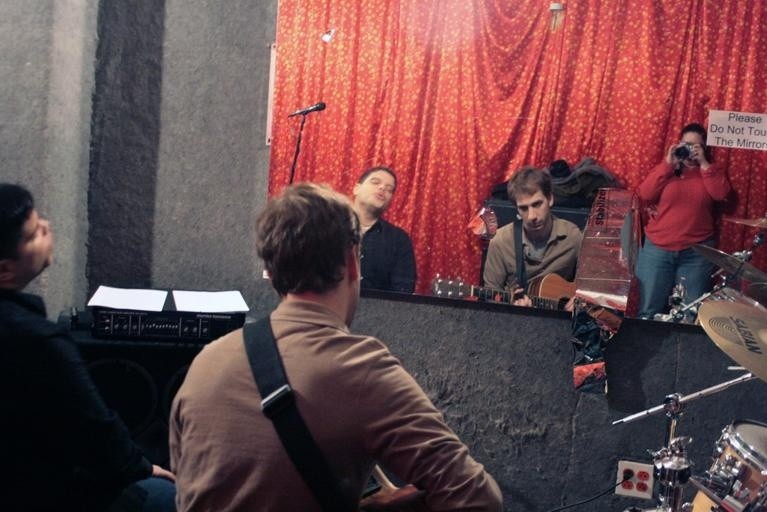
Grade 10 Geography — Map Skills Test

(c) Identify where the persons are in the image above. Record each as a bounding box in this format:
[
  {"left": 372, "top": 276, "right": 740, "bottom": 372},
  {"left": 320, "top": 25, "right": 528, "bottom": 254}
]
[
  {"left": 634, "top": 122, "right": 733, "bottom": 322},
  {"left": 168, "top": 180, "right": 503, "bottom": 512},
  {"left": 0, "top": 182, "right": 176, "bottom": 512},
  {"left": 483, "top": 166, "right": 584, "bottom": 293},
  {"left": 351, "top": 167, "right": 418, "bottom": 294}
]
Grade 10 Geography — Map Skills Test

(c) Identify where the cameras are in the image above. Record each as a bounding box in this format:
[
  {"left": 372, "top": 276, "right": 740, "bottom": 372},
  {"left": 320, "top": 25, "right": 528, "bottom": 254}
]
[{"left": 674, "top": 144, "right": 695, "bottom": 160}]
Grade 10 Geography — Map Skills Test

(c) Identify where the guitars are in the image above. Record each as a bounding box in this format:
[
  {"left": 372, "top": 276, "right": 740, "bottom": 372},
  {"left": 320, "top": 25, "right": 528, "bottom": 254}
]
[{"left": 431, "top": 274, "right": 575, "bottom": 312}]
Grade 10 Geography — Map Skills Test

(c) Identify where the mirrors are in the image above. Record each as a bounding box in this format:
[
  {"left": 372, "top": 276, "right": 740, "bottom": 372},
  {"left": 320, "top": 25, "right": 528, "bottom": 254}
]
[{"left": 267, "top": 1, "right": 767, "bottom": 328}]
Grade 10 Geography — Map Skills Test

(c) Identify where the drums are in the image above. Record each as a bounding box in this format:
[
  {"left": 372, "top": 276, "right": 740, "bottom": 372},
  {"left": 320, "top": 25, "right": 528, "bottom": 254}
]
[
  {"left": 707, "top": 287, "right": 767, "bottom": 311},
  {"left": 709, "top": 418, "right": 767, "bottom": 512}
]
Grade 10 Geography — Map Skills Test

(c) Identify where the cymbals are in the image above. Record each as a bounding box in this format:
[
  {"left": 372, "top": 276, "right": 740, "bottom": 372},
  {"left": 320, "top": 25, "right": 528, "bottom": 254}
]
[
  {"left": 724, "top": 217, "right": 767, "bottom": 229},
  {"left": 697, "top": 300, "right": 767, "bottom": 381},
  {"left": 691, "top": 243, "right": 767, "bottom": 284}
]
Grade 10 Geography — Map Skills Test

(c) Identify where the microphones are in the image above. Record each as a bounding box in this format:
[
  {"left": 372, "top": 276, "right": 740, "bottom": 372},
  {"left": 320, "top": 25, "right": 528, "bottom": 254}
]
[
  {"left": 744, "top": 230, "right": 765, "bottom": 263},
  {"left": 290, "top": 101, "right": 328, "bottom": 118}
]
